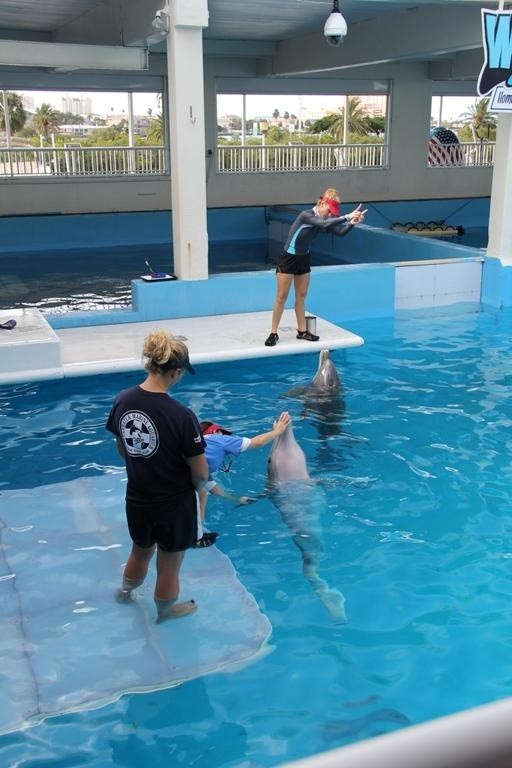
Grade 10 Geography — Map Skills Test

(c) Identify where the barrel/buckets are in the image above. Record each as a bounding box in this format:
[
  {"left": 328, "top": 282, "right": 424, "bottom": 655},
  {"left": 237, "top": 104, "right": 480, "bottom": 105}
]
[{"left": 305, "top": 315, "right": 316, "bottom": 336}]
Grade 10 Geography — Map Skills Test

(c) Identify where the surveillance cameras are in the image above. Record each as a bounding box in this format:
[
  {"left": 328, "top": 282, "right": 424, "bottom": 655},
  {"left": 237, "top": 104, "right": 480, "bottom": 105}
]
[{"left": 321, "top": 12, "right": 347, "bottom": 47}]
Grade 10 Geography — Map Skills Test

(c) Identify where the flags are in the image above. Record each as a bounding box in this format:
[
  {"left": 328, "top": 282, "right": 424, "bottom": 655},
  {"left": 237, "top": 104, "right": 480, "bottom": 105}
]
[{"left": 429, "top": 131, "right": 462, "bottom": 167}]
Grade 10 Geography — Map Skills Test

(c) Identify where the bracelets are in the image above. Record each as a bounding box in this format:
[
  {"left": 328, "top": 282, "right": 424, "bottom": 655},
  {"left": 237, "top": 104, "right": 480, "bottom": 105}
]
[{"left": 346, "top": 213, "right": 350, "bottom": 221}]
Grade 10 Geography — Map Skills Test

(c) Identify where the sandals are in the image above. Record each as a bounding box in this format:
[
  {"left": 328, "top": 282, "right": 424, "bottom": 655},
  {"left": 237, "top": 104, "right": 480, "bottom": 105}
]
[
  {"left": 296, "top": 331, "right": 319, "bottom": 340},
  {"left": 194, "top": 536, "right": 215, "bottom": 547},
  {"left": 265, "top": 333, "right": 279, "bottom": 345}
]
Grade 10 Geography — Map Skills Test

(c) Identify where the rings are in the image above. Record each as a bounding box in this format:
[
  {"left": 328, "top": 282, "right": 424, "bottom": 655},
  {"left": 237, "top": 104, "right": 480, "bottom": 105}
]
[{"left": 355, "top": 208, "right": 359, "bottom": 211}]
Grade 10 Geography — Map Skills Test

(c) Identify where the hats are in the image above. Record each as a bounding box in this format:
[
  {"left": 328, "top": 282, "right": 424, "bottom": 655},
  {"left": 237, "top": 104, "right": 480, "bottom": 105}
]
[
  {"left": 203, "top": 423, "right": 232, "bottom": 435},
  {"left": 173, "top": 356, "right": 195, "bottom": 375},
  {"left": 319, "top": 196, "right": 340, "bottom": 216}
]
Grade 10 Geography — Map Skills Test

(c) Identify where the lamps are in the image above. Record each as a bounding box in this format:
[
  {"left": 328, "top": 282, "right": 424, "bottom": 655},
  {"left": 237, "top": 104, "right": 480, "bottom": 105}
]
[{"left": 323, "top": 0, "right": 348, "bottom": 49}]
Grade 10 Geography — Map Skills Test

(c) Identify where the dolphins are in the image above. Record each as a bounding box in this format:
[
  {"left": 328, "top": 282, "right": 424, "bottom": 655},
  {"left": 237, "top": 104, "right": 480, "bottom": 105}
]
[
  {"left": 232, "top": 411, "right": 379, "bottom": 625},
  {"left": 281, "top": 349, "right": 347, "bottom": 477}
]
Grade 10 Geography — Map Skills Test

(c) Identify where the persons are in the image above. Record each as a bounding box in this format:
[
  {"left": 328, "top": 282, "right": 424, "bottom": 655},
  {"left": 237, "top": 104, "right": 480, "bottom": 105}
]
[
  {"left": 193, "top": 411, "right": 293, "bottom": 548},
  {"left": 104, "top": 329, "right": 209, "bottom": 624},
  {"left": 264, "top": 189, "right": 369, "bottom": 345}
]
